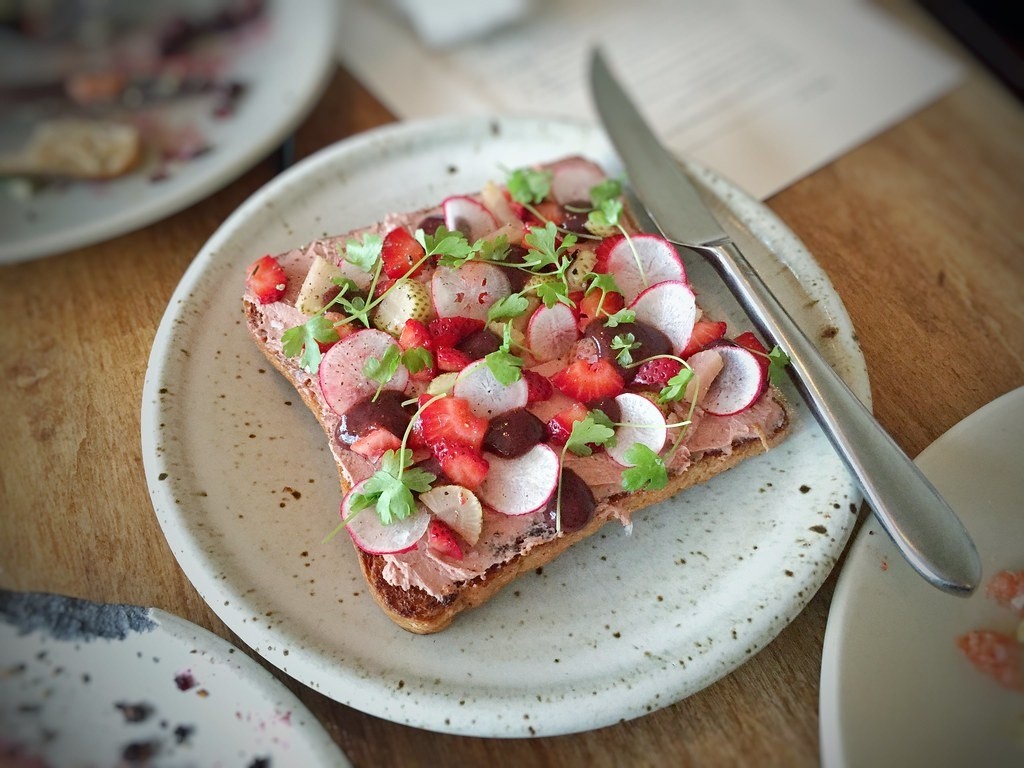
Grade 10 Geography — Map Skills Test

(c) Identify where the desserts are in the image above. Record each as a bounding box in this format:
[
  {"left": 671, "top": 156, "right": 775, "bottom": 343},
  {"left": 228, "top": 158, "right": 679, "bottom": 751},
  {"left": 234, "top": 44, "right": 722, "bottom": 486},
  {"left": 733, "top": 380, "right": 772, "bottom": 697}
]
[{"left": 240, "top": 155, "right": 796, "bottom": 635}]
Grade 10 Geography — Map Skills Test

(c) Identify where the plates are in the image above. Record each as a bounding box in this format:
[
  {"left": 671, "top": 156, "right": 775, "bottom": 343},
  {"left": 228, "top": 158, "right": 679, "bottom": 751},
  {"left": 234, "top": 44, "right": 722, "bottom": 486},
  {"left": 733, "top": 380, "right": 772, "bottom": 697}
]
[
  {"left": 1, "top": 586, "right": 354, "bottom": 768},
  {"left": 1, "top": 0, "right": 345, "bottom": 267},
  {"left": 817, "top": 381, "right": 1024, "bottom": 767},
  {"left": 137, "top": 108, "right": 875, "bottom": 742}
]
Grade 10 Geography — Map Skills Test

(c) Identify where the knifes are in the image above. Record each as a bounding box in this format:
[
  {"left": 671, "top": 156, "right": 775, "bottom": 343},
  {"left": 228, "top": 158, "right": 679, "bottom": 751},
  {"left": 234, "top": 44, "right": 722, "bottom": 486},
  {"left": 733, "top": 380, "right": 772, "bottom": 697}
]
[{"left": 584, "top": 43, "right": 986, "bottom": 596}]
